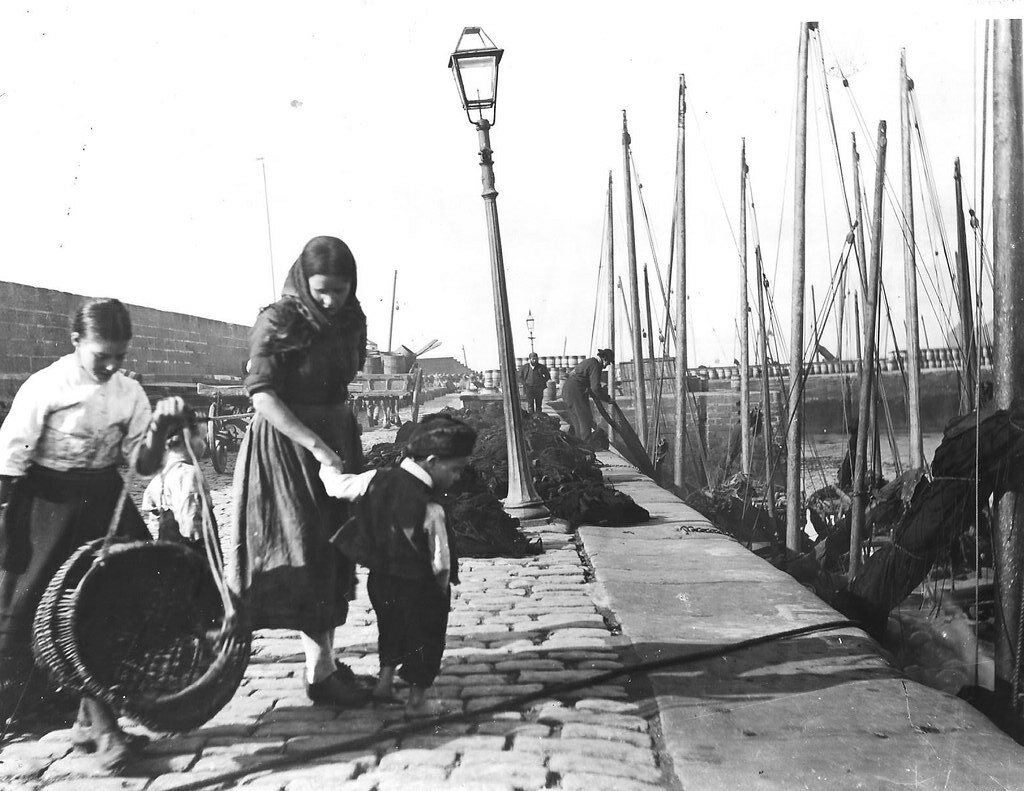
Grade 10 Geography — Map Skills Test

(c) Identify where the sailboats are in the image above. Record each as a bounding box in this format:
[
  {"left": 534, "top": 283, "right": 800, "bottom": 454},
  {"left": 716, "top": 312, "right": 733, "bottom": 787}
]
[{"left": 586, "top": 19, "right": 1022, "bottom": 750}]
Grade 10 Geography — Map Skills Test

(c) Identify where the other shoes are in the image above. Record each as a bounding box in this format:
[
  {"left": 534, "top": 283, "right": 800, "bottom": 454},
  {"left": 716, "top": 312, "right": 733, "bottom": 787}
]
[
  {"left": 97, "top": 730, "right": 129, "bottom": 769},
  {"left": 72, "top": 725, "right": 150, "bottom": 750},
  {"left": 302, "top": 658, "right": 372, "bottom": 709}
]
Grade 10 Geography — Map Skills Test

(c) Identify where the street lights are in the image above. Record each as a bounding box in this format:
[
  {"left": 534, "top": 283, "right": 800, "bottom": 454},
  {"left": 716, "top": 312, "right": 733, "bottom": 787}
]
[
  {"left": 524, "top": 309, "right": 535, "bottom": 354},
  {"left": 447, "top": 24, "right": 552, "bottom": 528}
]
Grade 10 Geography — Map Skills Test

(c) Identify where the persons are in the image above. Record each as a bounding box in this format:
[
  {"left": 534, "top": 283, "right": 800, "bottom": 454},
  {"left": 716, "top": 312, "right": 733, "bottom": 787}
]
[
  {"left": 222, "top": 236, "right": 381, "bottom": 707},
  {"left": 0, "top": 299, "right": 189, "bottom": 769},
  {"left": 562, "top": 348, "right": 615, "bottom": 442},
  {"left": 518, "top": 353, "right": 550, "bottom": 413},
  {"left": 140, "top": 412, "right": 227, "bottom": 567},
  {"left": 470, "top": 370, "right": 483, "bottom": 381},
  {"left": 318, "top": 414, "right": 477, "bottom": 716}
]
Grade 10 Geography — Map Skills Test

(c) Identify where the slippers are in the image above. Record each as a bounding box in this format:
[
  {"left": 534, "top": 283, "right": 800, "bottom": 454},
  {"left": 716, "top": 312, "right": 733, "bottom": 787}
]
[
  {"left": 404, "top": 700, "right": 452, "bottom": 722},
  {"left": 369, "top": 691, "right": 407, "bottom": 709}
]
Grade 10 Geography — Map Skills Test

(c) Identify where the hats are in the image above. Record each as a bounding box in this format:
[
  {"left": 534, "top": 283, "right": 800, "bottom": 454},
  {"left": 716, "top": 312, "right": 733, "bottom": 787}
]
[
  {"left": 404, "top": 412, "right": 476, "bottom": 459},
  {"left": 598, "top": 349, "right": 614, "bottom": 364}
]
